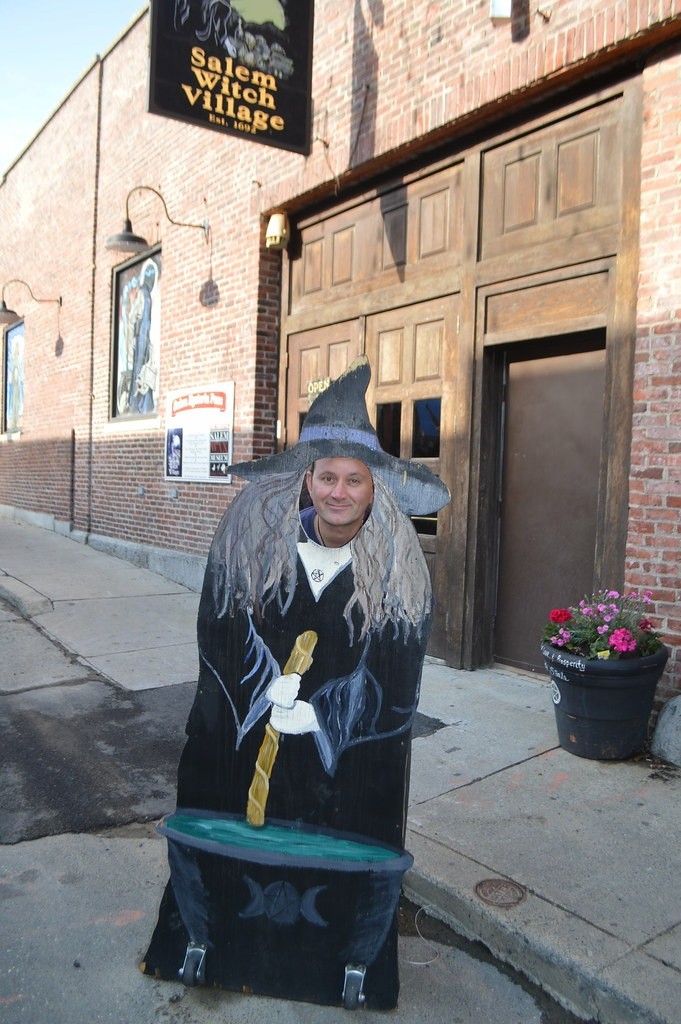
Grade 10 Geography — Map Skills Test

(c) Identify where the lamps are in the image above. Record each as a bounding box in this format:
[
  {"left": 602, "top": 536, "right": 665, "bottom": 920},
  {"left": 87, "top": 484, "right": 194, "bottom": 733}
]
[
  {"left": 105, "top": 186, "right": 206, "bottom": 253},
  {"left": 0, "top": 279, "right": 59, "bottom": 324}
]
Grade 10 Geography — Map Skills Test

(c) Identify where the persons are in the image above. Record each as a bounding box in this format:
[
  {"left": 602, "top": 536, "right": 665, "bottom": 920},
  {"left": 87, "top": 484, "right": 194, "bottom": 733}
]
[{"left": 299, "top": 456, "right": 374, "bottom": 548}]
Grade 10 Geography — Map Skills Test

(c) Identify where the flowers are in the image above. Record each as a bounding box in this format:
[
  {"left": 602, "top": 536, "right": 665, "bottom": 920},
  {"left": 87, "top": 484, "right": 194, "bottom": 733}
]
[{"left": 539, "top": 588, "right": 667, "bottom": 661}]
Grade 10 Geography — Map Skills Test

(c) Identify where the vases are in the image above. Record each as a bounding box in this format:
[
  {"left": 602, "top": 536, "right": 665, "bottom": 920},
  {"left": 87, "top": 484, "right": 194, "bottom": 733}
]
[{"left": 540, "top": 639, "right": 669, "bottom": 758}]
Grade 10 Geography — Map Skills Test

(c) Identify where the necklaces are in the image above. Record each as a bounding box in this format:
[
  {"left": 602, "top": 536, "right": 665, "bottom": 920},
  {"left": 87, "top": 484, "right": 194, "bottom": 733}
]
[{"left": 318, "top": 516, "right": 364, "bottom": 547}]
